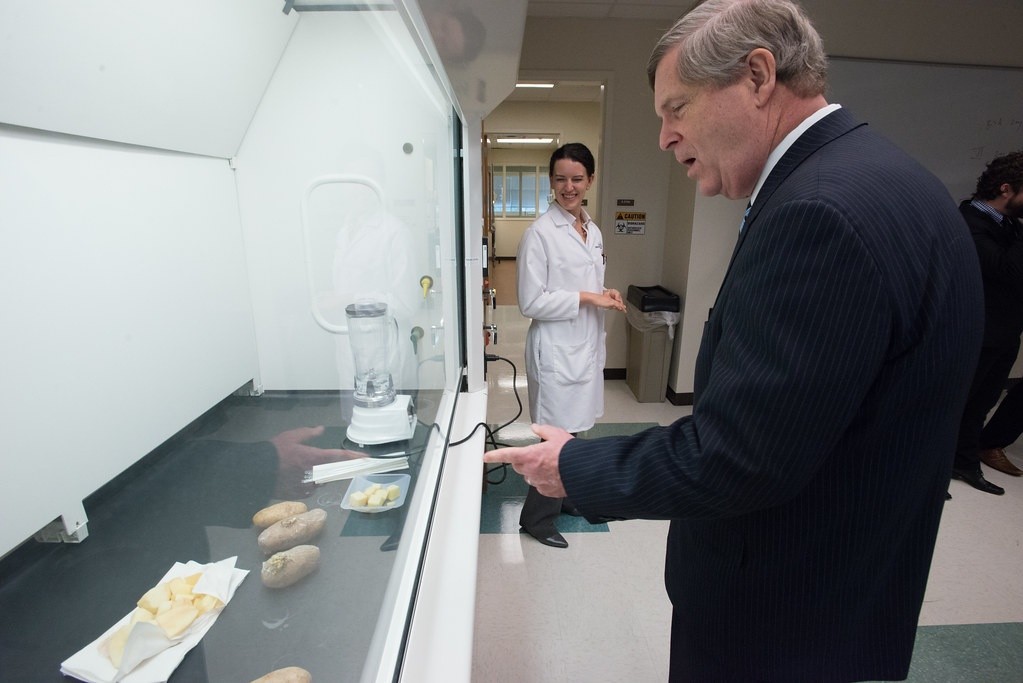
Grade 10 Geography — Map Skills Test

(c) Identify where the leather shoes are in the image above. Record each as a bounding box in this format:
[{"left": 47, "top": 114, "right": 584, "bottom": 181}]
[
  {"left": 560, "top": 502, "right": 581, "bottom": 517},
  {"left": 518, "top": 518, "right": 568, "bottom": 548}
]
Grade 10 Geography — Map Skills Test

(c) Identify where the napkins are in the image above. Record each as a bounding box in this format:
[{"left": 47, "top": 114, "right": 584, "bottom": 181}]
[{"left": 60, "top": 555, "right": 249, "bottom": 683}]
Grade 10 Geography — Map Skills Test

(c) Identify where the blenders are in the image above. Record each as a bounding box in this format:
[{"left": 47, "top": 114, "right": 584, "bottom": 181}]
[{"left": 346, "top": 293, "right": 418, "bottom": 445}]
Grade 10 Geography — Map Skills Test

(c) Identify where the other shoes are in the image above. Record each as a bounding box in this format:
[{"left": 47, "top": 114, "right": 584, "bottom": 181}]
[
  {"left": 951, "top": 471, "right": 1005, "bottom": 495},
  {"left": 978, "top": 445, "right": 1023, "bottom": 476}
]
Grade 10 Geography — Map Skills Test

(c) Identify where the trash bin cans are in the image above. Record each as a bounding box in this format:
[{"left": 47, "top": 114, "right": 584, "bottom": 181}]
[{"left": 626, "top": 284, "right": 681, "bottom": 403}]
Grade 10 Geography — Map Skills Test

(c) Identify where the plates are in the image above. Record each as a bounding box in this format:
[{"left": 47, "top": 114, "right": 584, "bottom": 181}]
[{"left": 340, "top": 473, "right": 411, "bottom": 513}]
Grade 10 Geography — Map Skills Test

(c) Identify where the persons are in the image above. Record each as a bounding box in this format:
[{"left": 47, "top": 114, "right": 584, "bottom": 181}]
[
  {"left": 484, "top": 0, "right": 1023, "bottom": 683},
  {"left": 517, "top": 141, "right": 627, "bottom": 548},
  {"left": 0, "top": 424, "right": 368, "bottom": 683}
]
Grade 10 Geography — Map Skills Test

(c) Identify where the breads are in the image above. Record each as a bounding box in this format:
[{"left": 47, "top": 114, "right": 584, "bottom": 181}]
[{"left": 251, "top": 666, "right": 312, "bottom": 683}]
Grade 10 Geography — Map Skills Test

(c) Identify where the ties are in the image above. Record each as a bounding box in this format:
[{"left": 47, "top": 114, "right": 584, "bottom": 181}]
[{"left": 739, "top": 206, "right": 752, "bottom": 234}]
